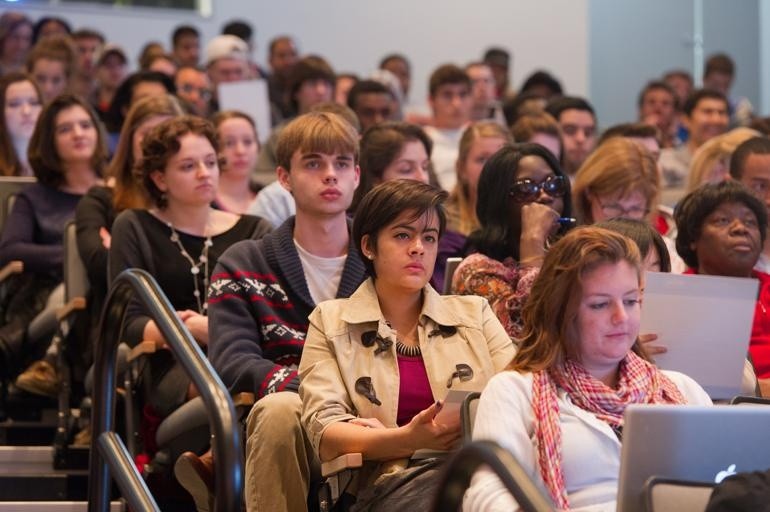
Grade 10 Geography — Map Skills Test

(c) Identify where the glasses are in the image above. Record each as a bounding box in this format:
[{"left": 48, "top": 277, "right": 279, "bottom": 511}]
[{"left": 509, "top": 175, "right": 566, "bottom": 203}]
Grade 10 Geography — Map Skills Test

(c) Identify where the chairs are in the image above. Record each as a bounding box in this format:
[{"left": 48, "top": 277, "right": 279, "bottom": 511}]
[
  {"left": 0, "top": 176, "right": 36, "bottom": 238},
  {"left": 64, "top": 222, "right": 89, "bottom": 309}
]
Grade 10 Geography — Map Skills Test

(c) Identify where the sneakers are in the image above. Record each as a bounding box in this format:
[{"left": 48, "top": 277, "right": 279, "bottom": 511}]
[{"left": 16, "top": 361, "right": 64, "bottom": 399}]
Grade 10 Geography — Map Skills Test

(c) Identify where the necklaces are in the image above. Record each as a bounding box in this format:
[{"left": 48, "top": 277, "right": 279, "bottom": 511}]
[
  {"left": 153, "top": 206, "right": 214, "bottom": 315},
  {"left": 386, "top": 321, "right": 421, "bottom": 357}
]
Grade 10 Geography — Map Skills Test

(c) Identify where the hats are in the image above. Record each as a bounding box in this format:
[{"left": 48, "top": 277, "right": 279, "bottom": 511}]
[{"left": 207, "top": 35, "right": 247, "bottom": 66}]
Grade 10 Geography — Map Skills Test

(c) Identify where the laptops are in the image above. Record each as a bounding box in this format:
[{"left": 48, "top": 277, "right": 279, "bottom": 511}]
[{"left": 613, "top": 405, "right": 769, "bottom": 511}]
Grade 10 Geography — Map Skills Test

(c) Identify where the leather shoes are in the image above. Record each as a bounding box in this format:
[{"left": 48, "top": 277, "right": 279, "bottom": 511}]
[{"left": 173, "top": 450, "right": 214, "bottom": 512}]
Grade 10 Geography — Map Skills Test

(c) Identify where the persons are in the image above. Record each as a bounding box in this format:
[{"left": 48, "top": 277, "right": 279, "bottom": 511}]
[
  {"left": 458, "top": 224, "right": 717, "bottom": 512},
  {"left": 706, "top": 462, "right": 770, "bottom": 512},
  {"left": 205, "top": 109, "right": 392, "bottom": 512},
  {"left": 105, "top": 117, "right": 281, "bottom": 512},
  {"left": 0, "top": 10, "right": 768, "bottom": 416},
  {"left": 295, "top": 177, "right": 518, "bottom": 512}
]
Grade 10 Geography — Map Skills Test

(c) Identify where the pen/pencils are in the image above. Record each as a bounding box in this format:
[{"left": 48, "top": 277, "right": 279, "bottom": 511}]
[{"left": 553, "top": 217, "right": 576, "bottom": 223}]
